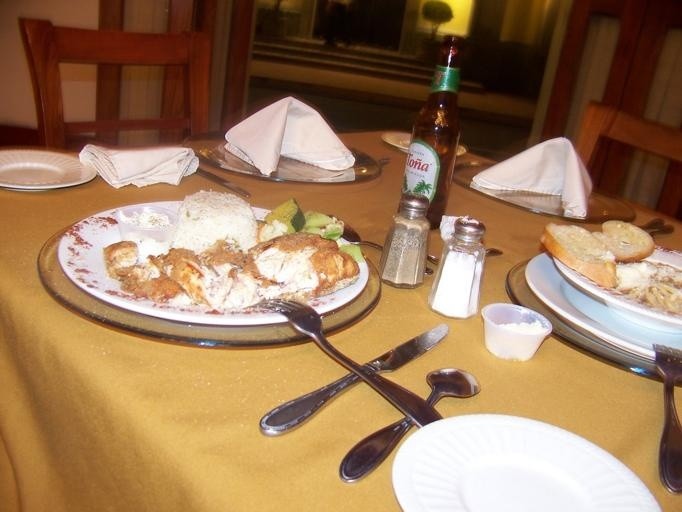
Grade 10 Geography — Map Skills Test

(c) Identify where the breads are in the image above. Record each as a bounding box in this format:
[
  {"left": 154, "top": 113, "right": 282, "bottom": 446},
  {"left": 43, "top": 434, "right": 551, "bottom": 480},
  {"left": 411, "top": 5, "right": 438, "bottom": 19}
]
[
  {"left": 539, "top": 222, "right": 617, "bottom": 289},
  {"left": 593, "top": 219, "right": 656, "bottom": 262}
]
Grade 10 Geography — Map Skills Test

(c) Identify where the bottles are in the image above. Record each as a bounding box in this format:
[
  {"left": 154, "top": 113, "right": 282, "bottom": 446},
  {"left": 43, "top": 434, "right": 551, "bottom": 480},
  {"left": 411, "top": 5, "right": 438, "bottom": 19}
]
[
  {"left": 394, "top": 36, "right": 463, "bottom": 231},
  {"left": 431, "top": 218, "right": 484, "bottom": 319},
  {"left": 380, "top": 191, "right": 431, "bottom": 287}
]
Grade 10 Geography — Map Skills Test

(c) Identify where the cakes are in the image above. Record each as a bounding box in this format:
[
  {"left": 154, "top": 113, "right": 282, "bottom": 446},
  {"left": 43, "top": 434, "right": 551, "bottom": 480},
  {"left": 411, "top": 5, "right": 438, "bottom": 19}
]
[{"left": 104, "top": 188, "right": 359, "bottom": 312}]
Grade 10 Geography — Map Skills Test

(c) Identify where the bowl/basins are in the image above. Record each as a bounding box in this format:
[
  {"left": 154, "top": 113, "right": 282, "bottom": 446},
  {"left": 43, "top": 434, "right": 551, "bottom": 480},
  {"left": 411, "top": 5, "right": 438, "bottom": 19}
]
[{"left": 482, "top": 302, "right": 552, "bottom": 361}]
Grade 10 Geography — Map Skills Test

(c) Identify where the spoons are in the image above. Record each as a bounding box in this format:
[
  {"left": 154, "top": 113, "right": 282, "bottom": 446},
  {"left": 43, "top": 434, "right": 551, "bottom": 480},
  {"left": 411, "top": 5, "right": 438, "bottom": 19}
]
[
  {"left": 327, "top": 215, "right": 435, "bottom": 276},
  {"left": 338, "top": 367, "right": 482, "bottom": 483}
]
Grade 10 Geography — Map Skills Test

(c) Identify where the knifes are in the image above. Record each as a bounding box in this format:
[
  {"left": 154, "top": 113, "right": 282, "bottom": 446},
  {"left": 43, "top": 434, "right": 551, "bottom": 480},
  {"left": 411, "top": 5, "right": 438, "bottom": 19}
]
[
  {"left": 192, "top": 168, "right": 250, "bottom": 196},
  {"left": 257, "top": 323, "right": 451, "bottom": 438}
]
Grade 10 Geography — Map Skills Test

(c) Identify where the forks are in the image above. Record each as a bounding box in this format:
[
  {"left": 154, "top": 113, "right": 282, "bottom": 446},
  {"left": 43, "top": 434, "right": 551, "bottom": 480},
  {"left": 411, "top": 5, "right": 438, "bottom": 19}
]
[
  {"left": 252, "top": 297, "right": 444, "bottom": 429},
  {"left": 652, "top": 344, "right": 682, "bottom": 496}
]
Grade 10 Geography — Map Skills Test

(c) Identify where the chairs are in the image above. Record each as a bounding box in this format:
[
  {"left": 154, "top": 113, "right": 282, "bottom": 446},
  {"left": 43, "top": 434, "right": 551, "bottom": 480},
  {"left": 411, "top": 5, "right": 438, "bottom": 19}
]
[
  {"left": 568, "top": 99, "right": 682, "bottom": 221},
  {"left": 18, "top": 14, "right": 214, "bottom": 149}
]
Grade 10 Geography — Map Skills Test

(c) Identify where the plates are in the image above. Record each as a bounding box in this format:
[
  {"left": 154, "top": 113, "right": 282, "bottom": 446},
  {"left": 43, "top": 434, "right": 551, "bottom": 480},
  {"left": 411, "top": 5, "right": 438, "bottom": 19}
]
[
  {"left": 525, "top": 250, "right": 682, "bottom": 365},
  {"left": 57, "top": 202, "right": 368, "bottom": 326},
  {"left": 393, "top": 415, "right": 663, "bottom": 512},
  {"left": 182, "top": 130, "right": 379, "bottom": 186},
  {"left": 0, "top": 146, "right": 97, "bottom": 193},
  {"left": 382, "top": 129, "right": 465, "bottom": 159},
  {"left": 552, "top": 254, "right": 682, "bottom": 336}
]
